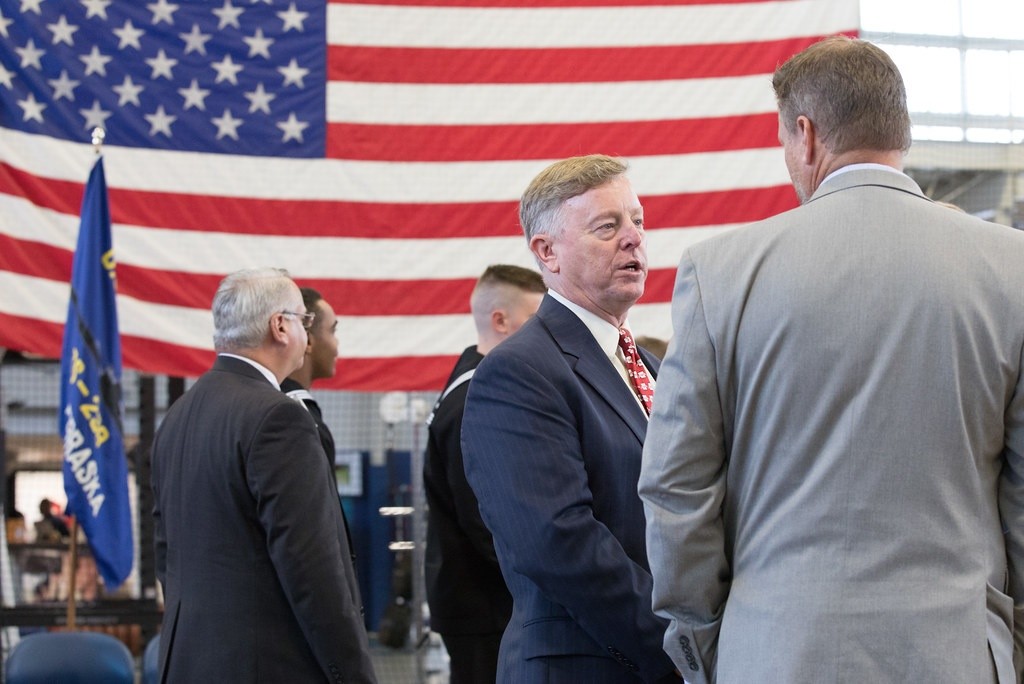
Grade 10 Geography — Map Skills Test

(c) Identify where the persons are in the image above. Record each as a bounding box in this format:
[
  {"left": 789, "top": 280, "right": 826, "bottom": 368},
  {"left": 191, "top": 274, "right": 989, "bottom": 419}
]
[
  {"left": 424, "top": 264, "right": 548, "bottom": 684},
  {"left": 1, "top": 267, "right": 380, "bottom": 683},
  {"left": 461, "top": 152, "right": 682, "bottom": 684},
  {"left": 638, "top": 38, "right": 1024, "bottom": 684}
]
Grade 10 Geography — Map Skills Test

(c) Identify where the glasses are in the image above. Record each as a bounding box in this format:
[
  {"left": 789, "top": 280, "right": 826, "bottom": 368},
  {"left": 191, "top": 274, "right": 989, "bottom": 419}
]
[{"left": 282, "top": 301, "right": 320, "bottom": 329}]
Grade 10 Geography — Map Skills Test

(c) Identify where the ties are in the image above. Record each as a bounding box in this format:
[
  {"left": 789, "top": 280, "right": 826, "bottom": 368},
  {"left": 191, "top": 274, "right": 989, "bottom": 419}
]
[{"left": 618, "top": 327, "right": 655, "bottom": 417}]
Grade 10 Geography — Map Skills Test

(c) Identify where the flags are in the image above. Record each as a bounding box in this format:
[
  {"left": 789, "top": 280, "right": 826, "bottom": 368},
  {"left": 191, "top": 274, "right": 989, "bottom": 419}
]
[
  {"left": 57, "top": 154, "right": 134, "bottom": 589},
  {"left": 0, "top": 1, "right": 861, "bottom": 392}
]
